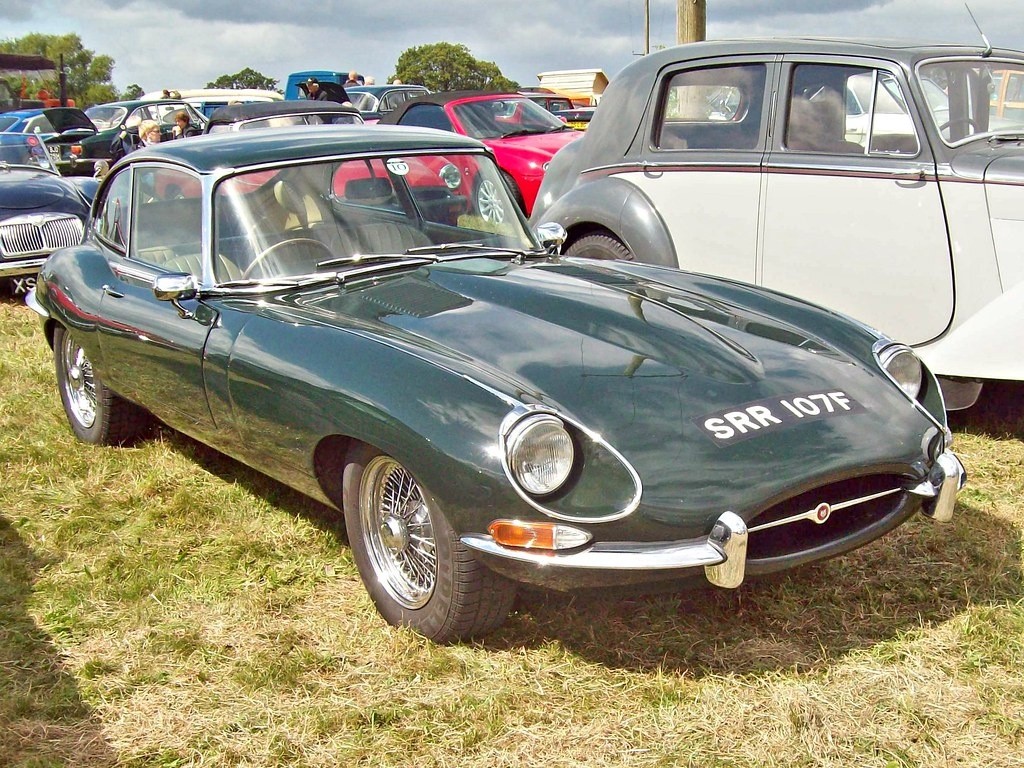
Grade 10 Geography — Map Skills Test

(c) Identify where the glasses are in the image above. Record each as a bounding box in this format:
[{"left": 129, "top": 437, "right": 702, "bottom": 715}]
[{"left": 150, "top": 130, "right": 161, "bottom": 133}]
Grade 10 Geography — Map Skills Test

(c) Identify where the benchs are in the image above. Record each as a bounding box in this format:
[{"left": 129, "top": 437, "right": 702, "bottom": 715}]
[
  {"left": 137, "top": 224, "right": 344, "bottom": 279},
  {"left": 659, "top": 125, "right": 759, "bottom": 149}
]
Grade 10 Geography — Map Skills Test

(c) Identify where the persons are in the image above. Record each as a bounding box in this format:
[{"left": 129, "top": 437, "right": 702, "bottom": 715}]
[
  {"left": 344, "top": 71, "right": 402, "bottom": 90},
  {"left": 160, "top": 90, "right": 181, "bottom": 100},
  {"left": 302, "top": 77, "right": 327, "bottom": 101},
  {"left": 111, "top": 115, "right": 162, "bottom": 162},
  {"left": 171, "top": 112, "right": 196, "bottom": 139}
]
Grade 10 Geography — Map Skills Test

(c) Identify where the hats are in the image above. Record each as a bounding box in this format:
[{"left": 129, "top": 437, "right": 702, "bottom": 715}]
[
  {"left": 366, "top": 77, "right": 375, "bottom": 83},
  {"left": 307, "top": 78, "right": 318, "bottom": 83},
  {"left": 349, "top": 71, "right": 357, "bottom": 78},
  {"left": 393, "top": 80, "right": 401, "bottom": 84}
]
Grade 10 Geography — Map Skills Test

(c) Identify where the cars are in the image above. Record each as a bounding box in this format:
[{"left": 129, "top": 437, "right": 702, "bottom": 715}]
[
  {"left": 524, "top": 35, "right": 1024, "bottom": 423},
  {"left": 0, "top": 70, "right": 607, "bottom": 294}
]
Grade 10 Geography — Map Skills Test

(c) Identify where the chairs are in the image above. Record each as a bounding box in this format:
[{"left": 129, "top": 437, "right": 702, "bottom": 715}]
[
  {"left": 161, "top": 252, "right": 252, "bottom": 283},
  {"left": 332, "top": 223, "right": 432, "bottom": 258},
  {"left": 786, "top": 101, "right": 864, "bottom": 153}
]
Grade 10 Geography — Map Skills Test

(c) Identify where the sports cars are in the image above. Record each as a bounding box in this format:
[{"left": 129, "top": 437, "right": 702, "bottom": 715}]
[{"left": 22, "top": 125, "right": 970, "bottom": 647}]
